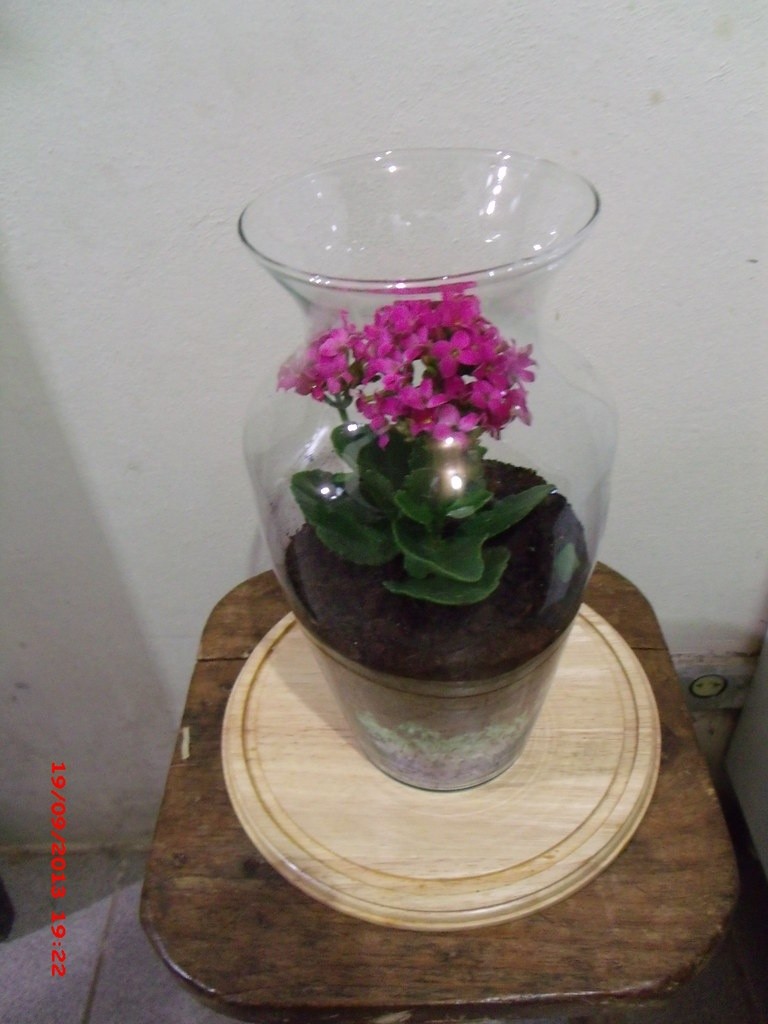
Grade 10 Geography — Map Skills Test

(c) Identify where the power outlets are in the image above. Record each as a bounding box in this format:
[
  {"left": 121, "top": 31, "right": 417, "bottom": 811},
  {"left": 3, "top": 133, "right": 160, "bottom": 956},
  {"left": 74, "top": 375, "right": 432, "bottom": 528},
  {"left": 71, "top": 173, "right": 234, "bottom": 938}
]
[{"left": 672, "top": 654, "right": 754, "bottom": 710}]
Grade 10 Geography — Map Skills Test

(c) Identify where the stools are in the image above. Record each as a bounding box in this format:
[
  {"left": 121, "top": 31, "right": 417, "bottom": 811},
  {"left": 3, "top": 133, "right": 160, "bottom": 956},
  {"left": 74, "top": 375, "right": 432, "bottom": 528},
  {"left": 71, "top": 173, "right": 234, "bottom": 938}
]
[{"left": 143, "top": 557, "right": 738, "bottom": 1023}]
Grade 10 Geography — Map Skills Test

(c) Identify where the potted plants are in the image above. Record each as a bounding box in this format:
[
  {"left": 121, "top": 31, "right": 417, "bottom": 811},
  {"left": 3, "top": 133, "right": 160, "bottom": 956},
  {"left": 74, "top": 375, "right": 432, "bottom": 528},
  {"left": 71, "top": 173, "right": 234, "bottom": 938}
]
[{"left": 236, "top": 149, "right": 623, "bottom": 792}]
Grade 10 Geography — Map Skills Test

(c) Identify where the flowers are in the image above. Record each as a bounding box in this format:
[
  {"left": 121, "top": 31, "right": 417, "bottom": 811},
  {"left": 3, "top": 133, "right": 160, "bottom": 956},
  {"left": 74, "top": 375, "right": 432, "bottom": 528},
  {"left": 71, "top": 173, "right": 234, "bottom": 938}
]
[{"left": 275, "top": 282, "right": 555, "bottom": 612}]
